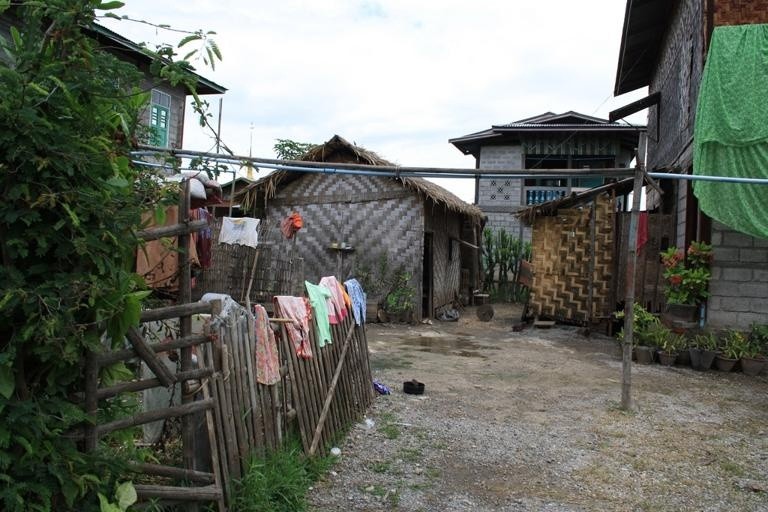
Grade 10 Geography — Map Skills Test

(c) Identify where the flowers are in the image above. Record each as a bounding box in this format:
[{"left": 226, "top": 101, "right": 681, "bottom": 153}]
[{"left": 658, "top": 241, "right": 714, "bottom": 305}]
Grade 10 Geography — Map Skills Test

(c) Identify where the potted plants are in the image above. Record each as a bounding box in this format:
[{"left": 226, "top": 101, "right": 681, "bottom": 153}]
[
  {"left": 611, "top": 302, "right": 768, "bottom": 373},
  {"left": 384, "top": 271, "right": 416, "bottom": 324}
]
